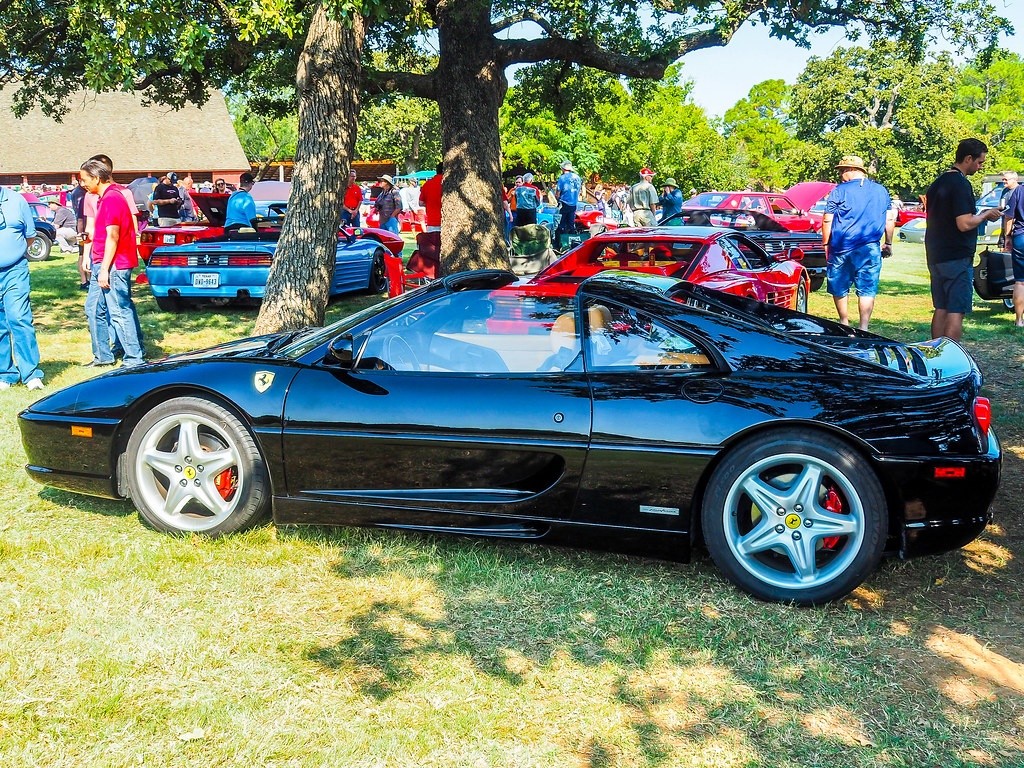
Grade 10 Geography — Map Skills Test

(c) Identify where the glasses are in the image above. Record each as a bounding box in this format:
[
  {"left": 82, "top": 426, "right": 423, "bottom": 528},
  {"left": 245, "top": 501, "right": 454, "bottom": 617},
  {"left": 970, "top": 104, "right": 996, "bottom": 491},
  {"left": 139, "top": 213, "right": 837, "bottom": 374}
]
[
  {"left": 1002, "top": 177, "right": 1016, "bottom": 181},
  {"left": 217, "top": 183, "right": 224, "bottom": 186}
]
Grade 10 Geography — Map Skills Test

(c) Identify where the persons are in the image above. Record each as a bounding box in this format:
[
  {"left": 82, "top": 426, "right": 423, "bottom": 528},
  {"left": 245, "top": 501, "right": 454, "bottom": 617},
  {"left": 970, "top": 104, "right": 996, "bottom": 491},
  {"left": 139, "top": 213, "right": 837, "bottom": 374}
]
[
  {"left": 925, "top": 137, "right": 1006, "bottom": 343},
  {"left": 998, "top": 171, "right": 1019, "bottom": 249},
  {"left": 1004, "top": 184, "right": 1024, "bottom": 327},
  {"left": 822, "top": 156, "right": 896, "bottom": 332},
  {"left": 340, "top": 162, "right": 445, "bottom": 236},
  {"left": 0, "top": 183, "right": 45, "bottom": 391},
  {"left": 503, "top": 159, "right": 684, "bottom": 249},
  {"left": 38, "top": 153, "right": 259, "bottom": 368}
]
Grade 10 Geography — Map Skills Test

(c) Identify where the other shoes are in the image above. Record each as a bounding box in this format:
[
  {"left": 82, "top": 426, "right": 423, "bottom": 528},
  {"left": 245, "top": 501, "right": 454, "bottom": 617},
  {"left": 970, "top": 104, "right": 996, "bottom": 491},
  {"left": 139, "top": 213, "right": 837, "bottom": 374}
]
[
  {"left": 81, "top": 282, "right": 90, "bottom": 289},
  {"left": 84, "top": 361, "right": 113, "bottom": 368},
  {"left": 25, "top": 378, "right": 44, "bottom": 391},
  {"left": 0, "top": 381, "right": 10, "bottom": 391}
]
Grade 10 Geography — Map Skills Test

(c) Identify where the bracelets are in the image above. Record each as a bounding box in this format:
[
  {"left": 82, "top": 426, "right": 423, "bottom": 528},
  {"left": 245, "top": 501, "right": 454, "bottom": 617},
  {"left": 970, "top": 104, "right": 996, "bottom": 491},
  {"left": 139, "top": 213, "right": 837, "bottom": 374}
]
[
  {"left": 823, "top": 243, "right": 829, "bottom": 246},
  {"left": 885, "top": 242, "right": 892, "bottom": 245}
]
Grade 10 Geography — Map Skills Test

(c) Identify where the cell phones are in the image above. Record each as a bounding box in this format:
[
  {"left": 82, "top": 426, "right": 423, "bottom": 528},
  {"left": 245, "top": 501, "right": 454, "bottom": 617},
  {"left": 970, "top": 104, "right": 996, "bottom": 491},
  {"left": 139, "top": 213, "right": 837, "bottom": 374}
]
[{"left": 1000, "top": 205, "right": 1010, "bottom": 213}]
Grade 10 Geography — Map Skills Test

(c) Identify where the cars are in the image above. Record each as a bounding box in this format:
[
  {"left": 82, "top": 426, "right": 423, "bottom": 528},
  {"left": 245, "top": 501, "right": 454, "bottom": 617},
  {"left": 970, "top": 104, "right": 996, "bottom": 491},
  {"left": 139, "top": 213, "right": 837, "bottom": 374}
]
[
  {"left": 529, "top": 203, "right": 664, "bottom": 239},
  {"left": 898, "top": 181, "right": 1012, "bottom": 246},
  {"left": 21, "top": 191, "right": 74, "bottom": 262},
  {"left": 145, "top": 215, "right": 396, "bottom": 313},
  {"left": 655, "top": 208, "right": 828, "bottom": 292},
  {"left": 18, "top": 265, "right": 1002, "bottom": 606},
  {"left": 137, "top": 203, "right": 404, "bottom": 270},
  {"left": 681, "top": 181, "right": 838, "bottom": 234},
  {"left": 483, "top": 226, "right": 812, "bottom": 335},
  {"left": 893, "top": 208, "right": 926, "bottom": 228}
]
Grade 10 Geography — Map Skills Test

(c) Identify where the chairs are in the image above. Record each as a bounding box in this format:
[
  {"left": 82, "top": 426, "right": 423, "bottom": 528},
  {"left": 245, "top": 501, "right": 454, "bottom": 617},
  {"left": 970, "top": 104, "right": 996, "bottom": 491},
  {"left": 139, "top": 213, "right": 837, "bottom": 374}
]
[
  {"left": 559, "top": 232, "right": 591, "bottom": 258},
  {"left": 507, "top": 224, "right": 556, "bottom": 276},
  {"left": 587, "top": 304, "right": 614, "bottom": 367},
  {"left": 383, "top": 252, "right": 429, "bottom": 299},
  {"left": 536, "top": 311, "right": 575, "bottom": 372},
  {"left": 642, "top": 245, "right": 674, "bottom": 261},
  {"left": 406, "top": 231, "right": 441, "bottom": 285},
  {"left": 238, "top": 228, "right": 255, "bottom": 233}
]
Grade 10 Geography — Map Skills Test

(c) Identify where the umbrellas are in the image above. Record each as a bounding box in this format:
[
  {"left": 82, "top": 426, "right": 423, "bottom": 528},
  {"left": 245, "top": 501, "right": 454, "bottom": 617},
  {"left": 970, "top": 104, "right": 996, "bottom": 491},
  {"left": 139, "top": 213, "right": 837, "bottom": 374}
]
[{"left": 126, "top": 177, "right": 158, "bottom": 212}]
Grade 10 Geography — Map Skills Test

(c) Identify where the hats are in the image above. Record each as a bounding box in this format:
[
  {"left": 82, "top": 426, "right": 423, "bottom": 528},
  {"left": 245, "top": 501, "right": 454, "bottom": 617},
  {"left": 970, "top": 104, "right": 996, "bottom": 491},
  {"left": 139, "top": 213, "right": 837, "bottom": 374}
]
[
  {"left": 560, "top": 161, "right": 573, "bottom": 171},
  {"left": 43, "top": 196, "right": 62, "bottom": 206},
  {"left": 377, "top": 174, "right": 394, "bottom": 186},
  {"left": 167, "top": 172, "right": 178, "bottom": 183},
  {"left": 660, "top": 178, "right": 679, "bottom": 187},
  {"left": 640, "top": 168, "right": 655, "bottom": 176},
  {"left": 834, "top": 156, "right": 867, "bottom": 173}
]
